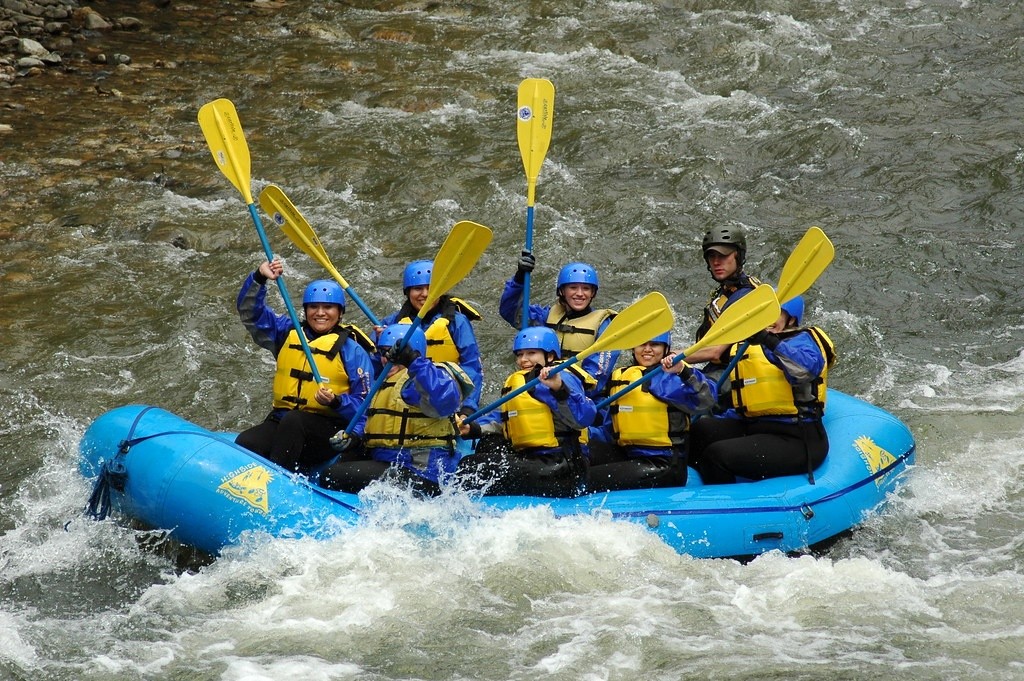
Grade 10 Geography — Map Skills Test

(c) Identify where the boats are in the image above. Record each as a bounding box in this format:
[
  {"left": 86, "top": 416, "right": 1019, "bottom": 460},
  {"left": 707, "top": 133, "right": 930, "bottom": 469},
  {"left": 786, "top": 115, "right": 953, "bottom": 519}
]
[{"left": 77, "top": 382, "right": 916, "bottom": 564}]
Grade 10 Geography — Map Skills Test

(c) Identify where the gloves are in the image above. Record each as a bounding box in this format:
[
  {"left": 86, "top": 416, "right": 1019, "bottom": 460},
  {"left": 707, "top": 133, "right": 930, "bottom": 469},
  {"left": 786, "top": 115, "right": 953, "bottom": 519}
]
[
  {"left": 385, "top": 338, "right": 419, "bottom": 369},
  {"left": 746, "top": 329, "right": 780, "bottom": 352},
  {"left": 328, "top": 430, "right": 361, "bottom": 453},
  {"left": 514, "top": 249, "right": 535, "bottom": 285}
]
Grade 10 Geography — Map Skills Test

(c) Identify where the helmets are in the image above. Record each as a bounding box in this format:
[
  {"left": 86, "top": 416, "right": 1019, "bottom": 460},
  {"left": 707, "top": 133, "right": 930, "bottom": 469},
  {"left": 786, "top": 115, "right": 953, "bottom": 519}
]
[
  {"left": 513, "top": 326, "right": 561, "bottom": 360},
  {"left": 302, "top": 279, "right": 345, "bottom": 315},
  {"left": 556, "top": 263, "right": 598, "bottom": 297},
  {"left": 702, "top": 224, "right": 747, "bottom": 255},
  {"left": 402, "top": 260, "right": 434, "bottom": 295},
  {"left": 649, "top": 330, "right": 671, "bottom": 346},
  {"left": 774, "top": 288, "right": 804, "bottom": 327},
  {"left": 378, "top": 324, "right": 427, "bottom": 359}
]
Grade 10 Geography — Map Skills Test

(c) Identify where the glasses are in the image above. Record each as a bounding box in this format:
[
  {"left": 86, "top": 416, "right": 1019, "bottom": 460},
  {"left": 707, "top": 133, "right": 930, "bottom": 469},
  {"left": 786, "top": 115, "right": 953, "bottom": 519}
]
[{"left": 381, "top": 348, "right": 390, "bottom": 357}]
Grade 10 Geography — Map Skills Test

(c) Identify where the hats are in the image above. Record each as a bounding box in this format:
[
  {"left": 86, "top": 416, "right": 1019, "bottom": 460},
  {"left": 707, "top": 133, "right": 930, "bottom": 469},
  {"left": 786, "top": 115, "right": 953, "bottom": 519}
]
[{"left": 705, "top": 244, "right": 738, "bottom": 255}]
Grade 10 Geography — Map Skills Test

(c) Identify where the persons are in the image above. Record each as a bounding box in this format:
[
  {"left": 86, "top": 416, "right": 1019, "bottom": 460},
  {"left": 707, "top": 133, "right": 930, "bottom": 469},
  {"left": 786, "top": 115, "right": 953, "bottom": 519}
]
[
  {"left": 367, "top": 259, "right": 482, "bottom": 420},
  {"left": 320, "top": 324, "right": 475, "bottom": 499},
  {"left": 672, "top": 224, "right": 762, "bottom": 394},
  {"left": 584, "top": 330, "right": 718, "bottom": 495},
  {"left": 686, "top": 287, "right": 837, "bottom": 484},
  {"left": 499, "top": 248, "right": 621, "bottom": 424},
  {"left": 457, "top": 327, "right": 597, "bottom": 499},
  {"left": 236, "top": 256, "right": 377, "bottom": 476}
]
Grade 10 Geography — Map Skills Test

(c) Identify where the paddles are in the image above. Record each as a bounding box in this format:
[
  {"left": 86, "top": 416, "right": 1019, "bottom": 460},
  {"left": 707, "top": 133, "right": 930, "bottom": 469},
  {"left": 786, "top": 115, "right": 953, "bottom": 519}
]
[
  {"left": 714, "top": 225, "right": 838, "bottom": 395},
  {"left": 596, "top": 283, "right": 782, "bottom": 409},
  {"left": 257, "top": 183, "right": 382, "bottom": 328},
  {"left": 514, "top": 77, "right": 558, "bottom": 329},
  {"left": 462, "top": 291, "right": 676, "bottom": 424},
  {"left": 196, "top": 97, "right": 329, "bottom": 392},
  {"left": 340, "top": 221, "right": 494, "bottom": 437}
]
